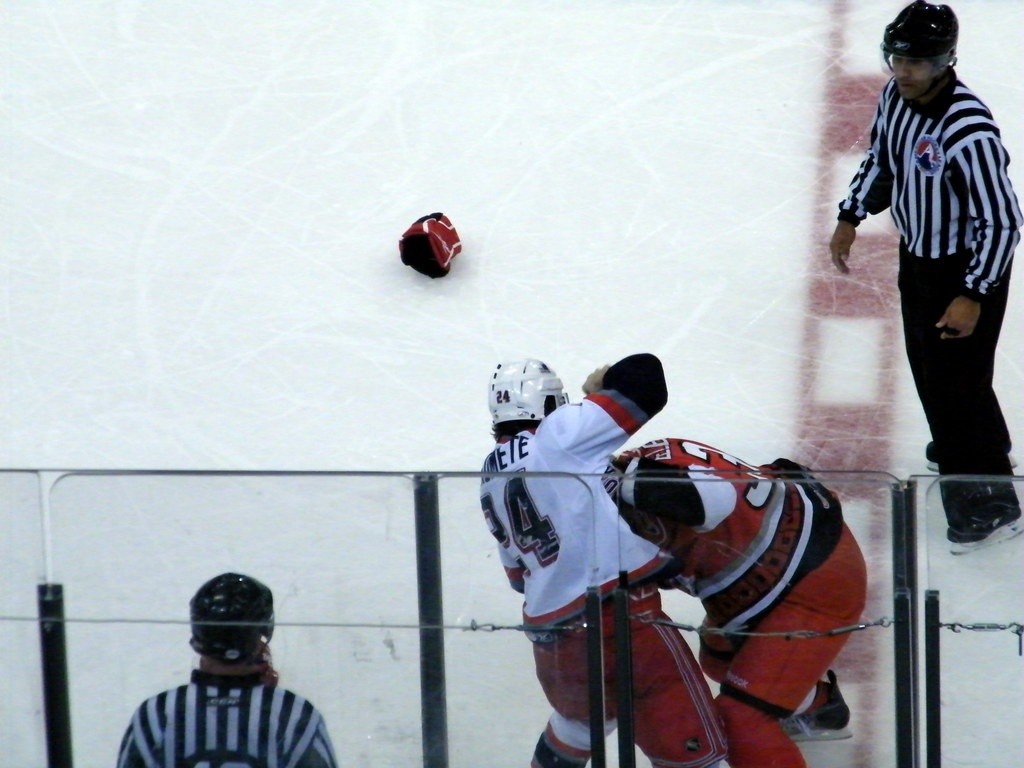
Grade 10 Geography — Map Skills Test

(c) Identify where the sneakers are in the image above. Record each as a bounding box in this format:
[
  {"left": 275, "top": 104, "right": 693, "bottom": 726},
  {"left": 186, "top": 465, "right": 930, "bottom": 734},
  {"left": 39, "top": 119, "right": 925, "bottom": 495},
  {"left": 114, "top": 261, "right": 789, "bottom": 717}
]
[
  {"left": 946, "top": 490, "right": 1021, "bottom": 553},
  {"left": 924, "top": 442, "right": 1016, "bottom": 474},
  {"left": 779, "top": 666, "right": 851, "bottom": 744}
]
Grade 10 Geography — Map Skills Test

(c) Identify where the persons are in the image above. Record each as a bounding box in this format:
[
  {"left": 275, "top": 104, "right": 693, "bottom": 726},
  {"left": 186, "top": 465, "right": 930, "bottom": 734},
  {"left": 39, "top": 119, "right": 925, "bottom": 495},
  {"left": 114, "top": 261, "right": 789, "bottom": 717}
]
[
  {"left": 601, "top": 439, "right": 868, "bottom": 768},
  {"left": 117, "top": 573, "right": 336, "bottom": 768},
  {"left": 829, "top": 0, "right": 1024, "bottom": 556},
  {"left": 479, "top": 354, "right": 729, "bottom": 768}
]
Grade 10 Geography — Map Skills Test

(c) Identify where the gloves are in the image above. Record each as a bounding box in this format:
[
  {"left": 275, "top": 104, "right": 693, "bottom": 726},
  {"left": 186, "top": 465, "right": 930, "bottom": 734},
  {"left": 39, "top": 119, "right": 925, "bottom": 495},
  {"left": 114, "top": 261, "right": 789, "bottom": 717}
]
[
  {"left": 582, "top": 365, "right": 611, "bottom": 395},
  {"left": 398, "top": 213, "right": 463, "bottom": 278}
]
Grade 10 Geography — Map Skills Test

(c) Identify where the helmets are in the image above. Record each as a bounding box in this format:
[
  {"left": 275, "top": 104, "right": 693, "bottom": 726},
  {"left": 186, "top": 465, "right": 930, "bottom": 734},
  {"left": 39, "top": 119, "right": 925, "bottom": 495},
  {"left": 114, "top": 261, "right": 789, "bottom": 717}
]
[
  {"left": 190, "top": 572, "right": 274, "bottom": 660},
  {"left": 488, "top": 359, "right": 564, "bottom": 426},
  {"left": 880, "top": 0, "right": 957, "bottom": 62}
]
